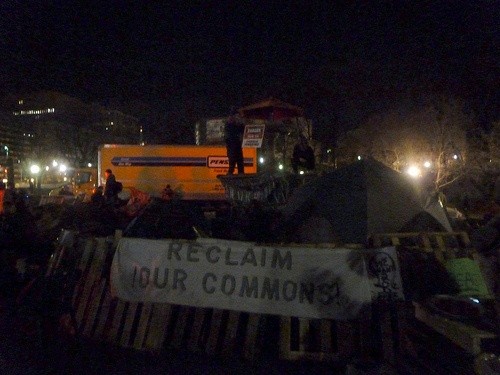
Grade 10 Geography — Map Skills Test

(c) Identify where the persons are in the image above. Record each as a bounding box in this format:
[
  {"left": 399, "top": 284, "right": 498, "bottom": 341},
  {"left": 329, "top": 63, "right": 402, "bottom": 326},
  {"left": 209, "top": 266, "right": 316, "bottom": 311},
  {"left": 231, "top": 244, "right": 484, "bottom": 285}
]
[
  {"left": 223, "top": 111, "right": 245, "bottom": 175},
  {"left": 0, "top": 168, "right": 282, "bottom": 247},
  {"left": 291, "top": 135, "right": 317, "bottom": 172}
]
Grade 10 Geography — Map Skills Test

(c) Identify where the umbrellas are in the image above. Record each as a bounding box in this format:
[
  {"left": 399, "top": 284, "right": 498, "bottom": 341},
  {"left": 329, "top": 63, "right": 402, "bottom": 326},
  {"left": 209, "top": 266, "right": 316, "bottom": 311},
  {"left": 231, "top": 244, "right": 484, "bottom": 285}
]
[{"left": 242, "top": 96, "right": 304, "bottom": 122}]
